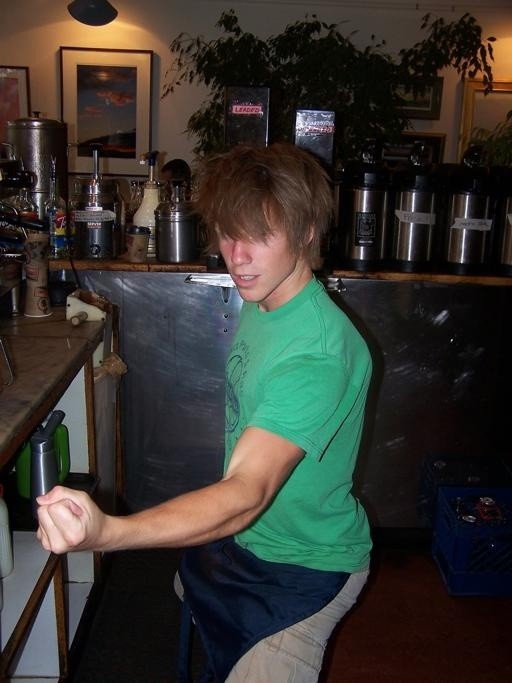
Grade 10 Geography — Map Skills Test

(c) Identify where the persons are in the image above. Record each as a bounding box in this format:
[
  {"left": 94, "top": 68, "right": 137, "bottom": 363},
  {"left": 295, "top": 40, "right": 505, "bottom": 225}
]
[{"left": 36, "top": 141, "right": 373, "bottom": 683}]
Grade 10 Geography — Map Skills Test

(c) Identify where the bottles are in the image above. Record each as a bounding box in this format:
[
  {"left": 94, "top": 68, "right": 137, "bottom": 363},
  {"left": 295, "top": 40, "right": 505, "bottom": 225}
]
[
  {"left": 0, "top": 137, "right": 187, "bottom": 262},
  {"left": 0, "top": 486, "right": 14, "bottom": 580}
]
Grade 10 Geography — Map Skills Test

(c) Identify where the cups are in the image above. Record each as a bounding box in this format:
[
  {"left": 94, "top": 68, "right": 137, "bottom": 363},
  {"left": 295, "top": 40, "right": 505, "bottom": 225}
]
[{"left": 14, "top": 422, "right": 72, "bottom": 505}]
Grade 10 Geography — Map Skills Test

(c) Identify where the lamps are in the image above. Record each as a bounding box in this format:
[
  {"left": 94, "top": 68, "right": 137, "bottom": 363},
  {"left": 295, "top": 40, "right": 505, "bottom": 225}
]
[{"left": 67, "top": 0, "right": 118, "bottom": 25}]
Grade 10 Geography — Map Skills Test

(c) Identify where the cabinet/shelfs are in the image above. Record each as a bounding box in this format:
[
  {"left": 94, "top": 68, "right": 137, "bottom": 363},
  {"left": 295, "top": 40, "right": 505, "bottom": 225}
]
[{"left": 0, "top": 307, "right": 127, "bottom": 683}]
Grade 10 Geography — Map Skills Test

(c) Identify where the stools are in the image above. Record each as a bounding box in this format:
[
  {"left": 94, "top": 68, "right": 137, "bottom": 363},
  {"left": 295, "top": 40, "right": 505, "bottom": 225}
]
[{"left": 173, "top": 571, "right": 214, "bottom": 682}]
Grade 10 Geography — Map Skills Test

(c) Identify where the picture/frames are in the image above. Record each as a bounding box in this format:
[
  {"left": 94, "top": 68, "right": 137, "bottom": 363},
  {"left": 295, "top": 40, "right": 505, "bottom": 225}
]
[
  {"left": 457, "top": 79, "right": 511, "bottom": 166},
  {"left": 1, "top": 65, "right": 31, "bottom": 164},
  {"left": 381, "top": 131, "right": 447, "bottom": 174},
  {"left": 60, "top": 46, "right": 153, "bottom": 178},
  {"left": 394, "top": 75, "right": 445, "bottom": 120}
]
